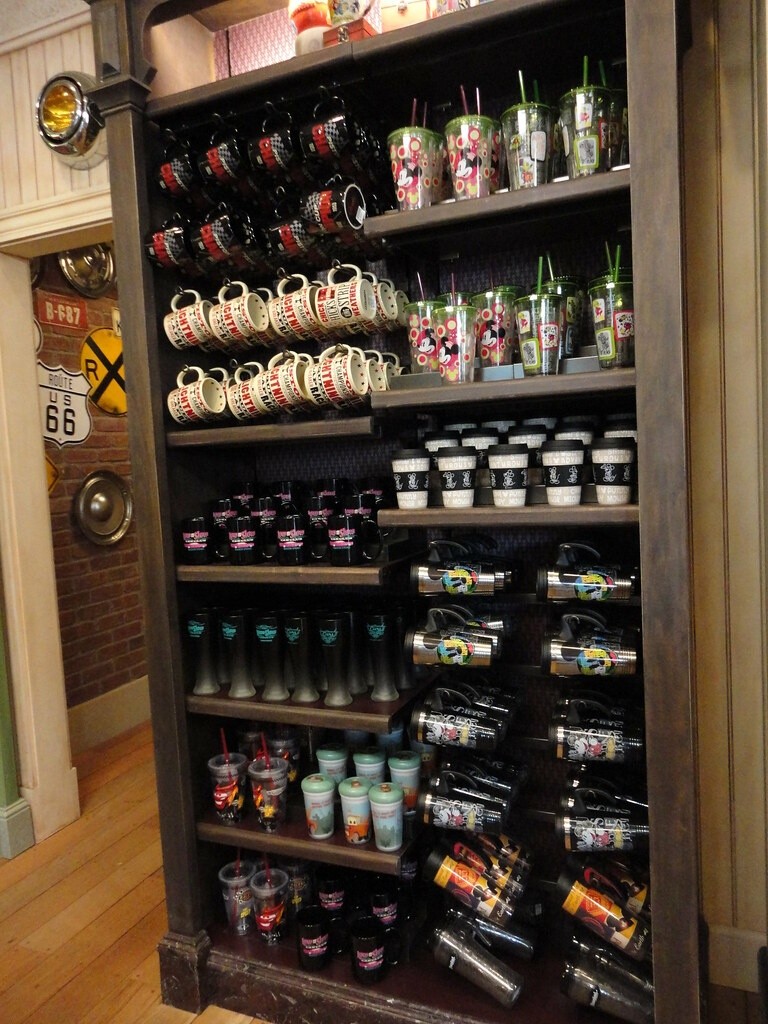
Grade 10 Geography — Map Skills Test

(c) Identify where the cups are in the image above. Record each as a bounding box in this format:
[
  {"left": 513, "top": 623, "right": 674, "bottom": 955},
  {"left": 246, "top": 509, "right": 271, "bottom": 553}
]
[{"left": 148, "top": 85, "right": 656, "bottom": 1024}]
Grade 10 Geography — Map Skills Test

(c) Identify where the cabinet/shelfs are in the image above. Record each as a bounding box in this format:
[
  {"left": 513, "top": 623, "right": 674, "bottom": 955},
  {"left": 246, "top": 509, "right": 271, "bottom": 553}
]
[{"left": 81, "top": 0, "right": 711, "bottom": 1024}]
